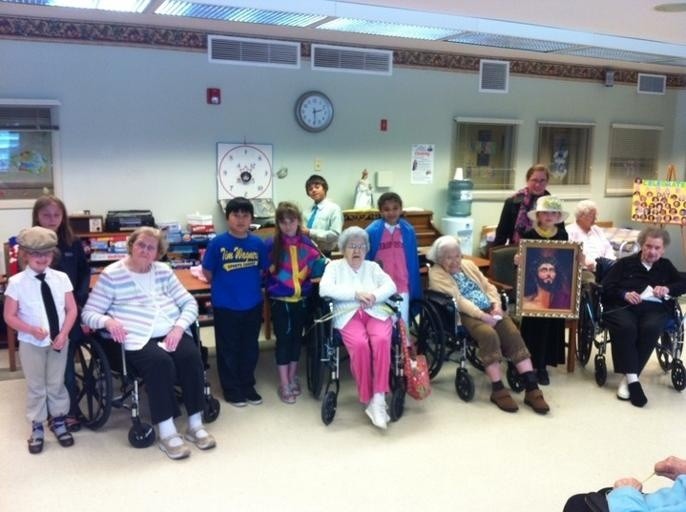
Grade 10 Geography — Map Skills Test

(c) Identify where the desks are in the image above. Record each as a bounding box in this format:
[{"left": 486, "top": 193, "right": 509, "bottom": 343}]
[{"left": 8, "top": 246, "right": 491, "bottom": 370}]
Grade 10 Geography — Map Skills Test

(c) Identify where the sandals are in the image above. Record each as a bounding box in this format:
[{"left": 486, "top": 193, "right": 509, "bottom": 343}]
[
  {"left": 290, "top": 383, "right": 300, "bottom": 396},
  {"left": 278, "top": 384, "right": 295, "bottom": 404}
]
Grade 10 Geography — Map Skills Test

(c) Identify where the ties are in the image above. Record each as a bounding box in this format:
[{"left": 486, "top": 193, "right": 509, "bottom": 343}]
[
  {"left": 307, "top": 206, "right": 318, "bottom": 229},
  {"left": 34, "top": 273, "right": 61, "bottom": 353}
]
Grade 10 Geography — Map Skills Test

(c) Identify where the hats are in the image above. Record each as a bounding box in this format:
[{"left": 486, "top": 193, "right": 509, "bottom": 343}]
[
  {"left": 527, "top": 195, "right": 569, "bottom": 224},
  {"left": 17, "top": 225, "right": 58, "bottom": 252}
]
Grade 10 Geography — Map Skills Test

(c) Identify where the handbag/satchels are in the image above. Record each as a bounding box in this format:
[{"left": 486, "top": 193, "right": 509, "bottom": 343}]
[{"left": 398, "top": 317, "right": 431, "bottom": 400}]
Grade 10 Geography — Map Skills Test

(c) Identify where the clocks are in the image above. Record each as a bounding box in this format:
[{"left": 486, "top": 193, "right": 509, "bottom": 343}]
[{"left": 295, "top": 91, "right": 335, "bottom": 133}]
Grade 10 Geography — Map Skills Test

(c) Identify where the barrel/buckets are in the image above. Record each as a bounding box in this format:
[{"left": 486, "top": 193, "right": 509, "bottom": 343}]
[{"left": 446, "top": 180, "right": 474, "bottom": 217}]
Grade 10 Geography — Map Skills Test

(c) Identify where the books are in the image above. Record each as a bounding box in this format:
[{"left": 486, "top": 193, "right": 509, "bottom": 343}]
[{"left": 87, "top": 236, "right": 130, "bottom": 261}]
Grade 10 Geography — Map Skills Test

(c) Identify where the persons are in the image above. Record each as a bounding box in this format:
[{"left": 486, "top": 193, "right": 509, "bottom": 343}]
[
  {"left": 32, "top": 196, "right": 91, "bottom": 432},
  {"left": 4, "top": 226, "right": 77, "bottom": 454},
  {"left": 427, "top": 235, "right": 549, "bottom": 415},
  {"left": 492, "top": 164, "right": 566, "bottom": 247},
  {"left": 364, "top": 192, "right": 421, "bottom": 357},
  {"left": 562, "top": 456, "right": 686, "bottom": 512},
  {"left": 260, "top": 202, "right": 320, "bottom": 403},
  {"left": 513, "top": 194, "right": 586, "bottom": 385},
  {"left": 202, "top": 196, "right": 273, "bottom": 407},
  {"left": 599, "top": 228, "right": 685, "bottom": 407},
  {"left": 354, "top": 169, "right": 374, "bottom": 209},
  {"left": 565, "top": 199, "right": 618, "bottom": 269},
  {"left": 299, "top": 174, "right": 344, "bottom": 251},
  {"left": 81, "top": 227, "right": 216, "bottom": 459},
  {"left": 318, "top": 225, "right": 397, "bottom": 431},
  {"left": 523, "top": 256, "right": 570, "bottom": 310}
]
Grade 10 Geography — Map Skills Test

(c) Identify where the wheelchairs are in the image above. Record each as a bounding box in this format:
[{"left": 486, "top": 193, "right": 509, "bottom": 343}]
[
  {"left": 305, "top": 283, "right": 408, "bottom": 425},
  {"left": 409, "top": 262, "right": 526, "bottom": 401},
  {"left": 74, "top": 305, "right": 221, "bottom": 449},
  {"left": 575, "top": 265, "right": 686, "bottom": 391}
]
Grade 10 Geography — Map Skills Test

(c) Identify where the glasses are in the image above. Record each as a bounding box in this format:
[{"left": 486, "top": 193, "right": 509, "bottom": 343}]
[
  {"left": 28, "top": 252, "right": 50, "bottom": 256},
  {"left": 529, "top": 178, "right": 547, "bottom": 182},
  {"left": 347, "top": 243, "right": 366, "bottom": 249}
]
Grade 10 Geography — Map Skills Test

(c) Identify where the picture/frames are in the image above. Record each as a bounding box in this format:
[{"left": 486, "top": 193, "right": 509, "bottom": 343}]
[
  {"left": 0, "top": 98, "right": 61, "bottom": 209},
  {"left": 516, "top": 239, "right": 584, "bottom": 320},
  {"left": 605, "top": 122, "right": 664, "bottom": 197},
  {"left": 450, "top": 116, "right": 524, "bottom": 202},
  {"left": 533, "top": 119, "right": 598, "bottom": 200}
]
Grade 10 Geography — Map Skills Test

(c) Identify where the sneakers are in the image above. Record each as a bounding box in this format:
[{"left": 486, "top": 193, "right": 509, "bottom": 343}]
[
  {"left": 28, "top": 421, "right": 44, "bottom": 453},
  {"left": 490, "top": 388, "right": 518, "bottom": 412},
  {"left": 365, "top": 398, "right": 387, "bottom": 430},
  {"left": 524, "top": 388, "right": 549, "bottom": 412},
  {"left": 226, "top": 400, "right": 246, "bottom": 407},
  {"left": 618, "top": 377, "right": 630, "bottom": 400},
  {"left": 48, "top": 414, "right": 53, "bottom": 432},
  {"left": 246, "top": 391, "right": 262, "bottom": 405},
  {"left": 48, "top": 416, "right": 74, "bottom": 447},
  {"left": 65, "top": 413, "right": 81, "bottom": 432},
  {"left": 184, "top": 425, "right": 216, "bottom": 450},
  {"left": 157, "top": 433, "right": 190, "bottom": 459}
]
